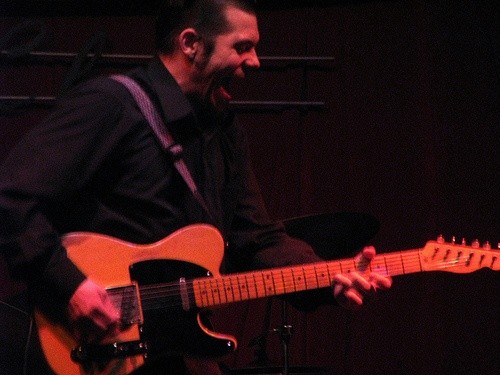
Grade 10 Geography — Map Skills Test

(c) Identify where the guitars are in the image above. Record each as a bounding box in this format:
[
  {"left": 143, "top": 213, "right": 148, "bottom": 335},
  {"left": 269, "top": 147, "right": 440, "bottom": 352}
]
[{"left": 36, "top": 222, "right": 499, "bottom": 375}]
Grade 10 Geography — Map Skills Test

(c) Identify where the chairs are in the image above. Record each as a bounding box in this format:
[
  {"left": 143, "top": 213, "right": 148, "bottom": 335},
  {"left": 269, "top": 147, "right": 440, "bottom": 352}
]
[{"left": 247, "top": 213, "right": 380, "bottom": 375}]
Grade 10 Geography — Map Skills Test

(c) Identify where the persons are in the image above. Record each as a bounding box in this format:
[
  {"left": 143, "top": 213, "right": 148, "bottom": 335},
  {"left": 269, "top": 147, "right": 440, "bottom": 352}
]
[{"left": 1, "top": 0, "right": 395, "bottom": 375}]
